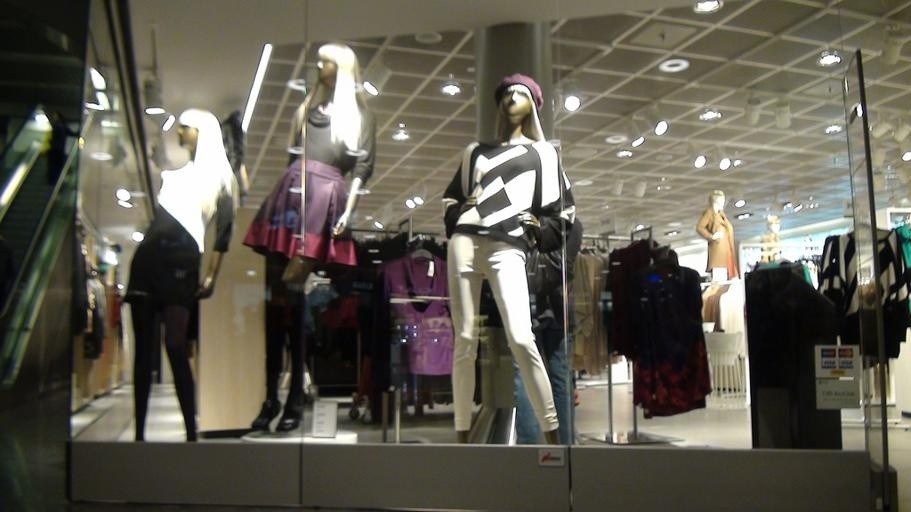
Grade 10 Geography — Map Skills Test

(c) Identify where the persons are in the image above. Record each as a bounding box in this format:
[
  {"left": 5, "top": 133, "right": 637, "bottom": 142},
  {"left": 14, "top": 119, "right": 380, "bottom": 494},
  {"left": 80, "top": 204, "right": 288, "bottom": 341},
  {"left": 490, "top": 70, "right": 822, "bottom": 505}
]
[
  {"left": 123, "top": 109, "right": 237, "bottom": 442},
  {"left": 441, "top": 73, "right": 576, "bottom": 444},
  {"left": 241, "top": 42, "right": 377, "bottom": 433},
  {"left": 514, "top": 215, "right": 582, "bottom": 445},
  {"left": 696, "top": 190, "right": 736, "bottom": 281}
]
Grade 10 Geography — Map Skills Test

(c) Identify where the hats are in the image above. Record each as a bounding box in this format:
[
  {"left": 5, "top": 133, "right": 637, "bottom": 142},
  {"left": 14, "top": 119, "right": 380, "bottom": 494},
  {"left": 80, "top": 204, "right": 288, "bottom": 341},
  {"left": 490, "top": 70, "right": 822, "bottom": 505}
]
[{"left": 494, "top": 73, "right": 543, "bottom": 113}]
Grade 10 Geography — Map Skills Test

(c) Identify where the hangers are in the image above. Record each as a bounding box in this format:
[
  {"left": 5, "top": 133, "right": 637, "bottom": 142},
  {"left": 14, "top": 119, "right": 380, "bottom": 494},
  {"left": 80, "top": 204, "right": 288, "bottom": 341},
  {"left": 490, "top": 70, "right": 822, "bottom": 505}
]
[
  {"left": 569, "top": 225, "right": 688, "bottom": 252},
  {"left": 742, "top": 238, "right": 828, "bottom": 290},
  {"left": 344, "top": 216, "right": 452, "bottom": 260}
]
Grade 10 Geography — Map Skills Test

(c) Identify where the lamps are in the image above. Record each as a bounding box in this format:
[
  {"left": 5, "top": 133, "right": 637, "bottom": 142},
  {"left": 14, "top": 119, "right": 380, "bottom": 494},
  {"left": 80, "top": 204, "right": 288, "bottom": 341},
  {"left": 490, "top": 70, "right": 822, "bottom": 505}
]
[
  {"left": 695, "top": 0, "right": 724, "bottom": 15},
  {"left": 392, "top": 122, "right": 410, "bottom": 141},
  {"left": 818, "top": 46, "right": 842, "bottom": 70},
  {"left": 443, "top": 70, "right": 460, "bottom": 97},
  {"left": 563, "top": 79, "right": 580, "bottom": 112},
  {"left": 716, "top": 147, "right": 732, "bottom": 172},
  {"left": 743, "top": 87, "right": 762, "bottom": 129},
  {"left": 894, "top": 165, "right": 910, "bottom": 187},
  {"left": 777, "top": 87, "right": 794, "bottom": 130},
  {"left": 147, "top": 79, "right": 165, "bottom": 119},
  {"left": 415, "top": 185, "right": 430, "bottom": 206},
  {"left": 790, "top": 186, "right": 801, "bottom": 212},
  {"left": 158, "top": 111, "right": 179, "bottom": 131},
  {"left": 874, "top": 144, "right": 888, "bottom": 166},
  {"left": 611, "top": 174, "right": 626, "bottom": 197},
  {"left": 645, "top": 106, "right": 668, "bottom": 137},
  {"left": 892, "top": 117, "right": 911, "bottom": 141},
  {"left": 417, "top": 30, "right": 442, "bottom": 45},
  {"left": 870, "top": 111, "right": 892, "bottom": 138},
  {"left": 879, "top": 24, "right": 910, "bottom": 69},
  {"left": 688, "top": 142, "right": 707, "bottom": 170},
  {"left": 623, "top": 115, "right": 646, "bottom": 149},
  {"left": 767, "top": 192, "right": 784, "bottom": 222},
  {"left": 734, "top": 187, "right": 745, "bottom": 208},
  {"left": 362, "top": 63, "right": 394, "bottom": 95},
  {"left": 699, "top": 101, "right": 724, "bottom": 123},
  {"left": 406, "top": 195, "right": 417, "bottom": 209},
  {"left": 633, "top": 177, "right": 646, "bottom": 201},
  {"left": 900, "top": 143, "right": 910, "bottom": 162}
]
